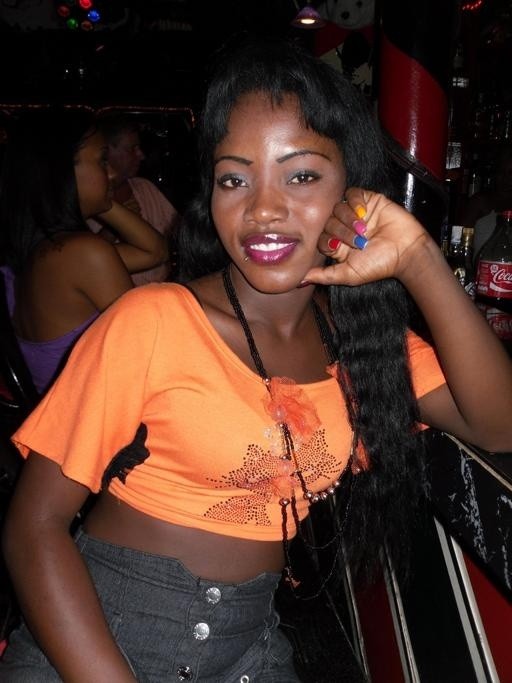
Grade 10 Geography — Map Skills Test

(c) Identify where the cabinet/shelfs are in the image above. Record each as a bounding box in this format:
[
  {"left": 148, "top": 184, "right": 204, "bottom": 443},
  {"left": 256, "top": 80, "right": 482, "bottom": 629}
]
[
  {"left": 441, "top": 221, "right": 475, "bottom": 297},
  {"left": 472, "top": 209, "right": 512, "bottom": 313}
]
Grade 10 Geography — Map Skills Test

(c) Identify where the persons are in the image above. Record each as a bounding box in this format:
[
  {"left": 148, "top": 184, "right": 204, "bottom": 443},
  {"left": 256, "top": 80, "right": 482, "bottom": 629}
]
[
  {"left": 448, "top": 148, "right": 511, "bottom": 261},
  {"left": 0, "top": 107, "right": 167, "bottom": 390},
  {"left": 0, "top": 46, "right": 512, "bottom": 680},
  {"left": 86, "top": 110, "right": 190, "bottom": 286}
]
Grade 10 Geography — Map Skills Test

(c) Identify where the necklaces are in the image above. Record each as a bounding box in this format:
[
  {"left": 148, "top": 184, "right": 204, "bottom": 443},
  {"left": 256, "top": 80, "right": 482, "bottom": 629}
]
[{"left": 221, "top": 265, "right": 363, "bottom": 601}]
